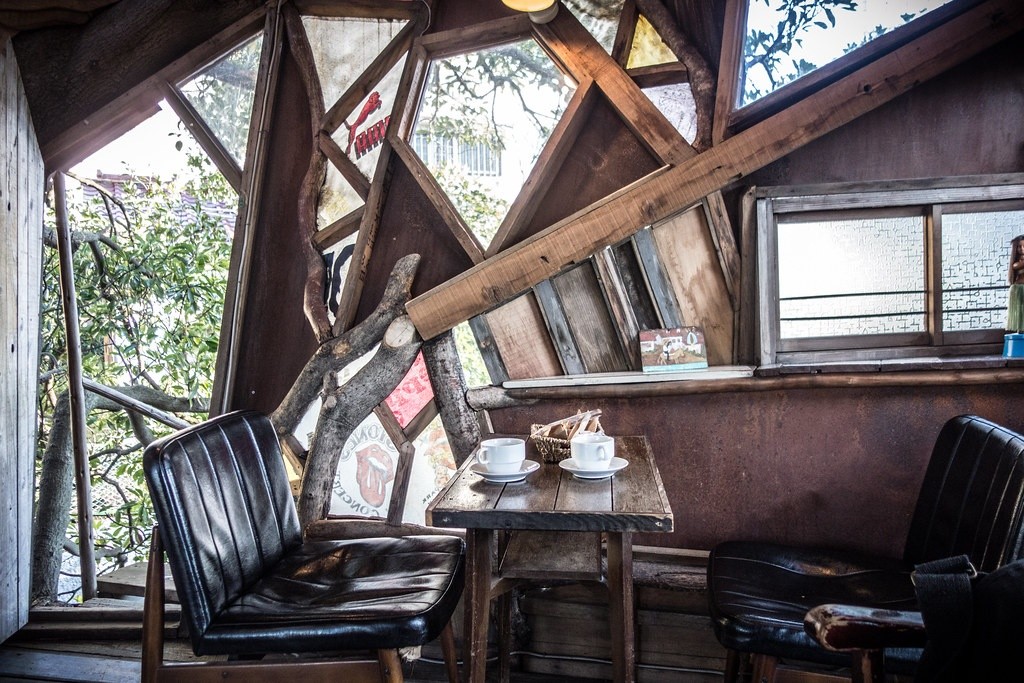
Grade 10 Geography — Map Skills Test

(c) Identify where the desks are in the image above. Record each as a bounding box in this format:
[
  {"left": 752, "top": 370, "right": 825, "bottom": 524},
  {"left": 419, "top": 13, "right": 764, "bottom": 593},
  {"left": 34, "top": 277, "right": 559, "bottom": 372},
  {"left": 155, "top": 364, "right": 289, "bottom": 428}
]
[{"left": 425, "top": 432, "right": 673, "bottom": 680}]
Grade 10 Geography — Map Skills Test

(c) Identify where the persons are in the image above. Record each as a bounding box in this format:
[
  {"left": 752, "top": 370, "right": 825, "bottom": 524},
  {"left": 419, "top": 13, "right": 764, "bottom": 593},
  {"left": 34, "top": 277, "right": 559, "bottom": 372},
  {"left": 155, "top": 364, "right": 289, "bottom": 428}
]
[{"left": 1005, "top": 234, "right": 1024, "bottom": 332}]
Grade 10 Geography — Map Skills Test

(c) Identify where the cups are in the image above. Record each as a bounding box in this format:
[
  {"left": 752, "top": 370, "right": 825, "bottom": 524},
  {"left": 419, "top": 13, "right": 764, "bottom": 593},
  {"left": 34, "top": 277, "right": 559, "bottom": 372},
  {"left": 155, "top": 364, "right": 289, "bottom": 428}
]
[
  {"left": 569, "top": 434, "right": 615, "bottom": 471},
  {"left": 477, "top": 437, "right": 527, "bottom": 475}
]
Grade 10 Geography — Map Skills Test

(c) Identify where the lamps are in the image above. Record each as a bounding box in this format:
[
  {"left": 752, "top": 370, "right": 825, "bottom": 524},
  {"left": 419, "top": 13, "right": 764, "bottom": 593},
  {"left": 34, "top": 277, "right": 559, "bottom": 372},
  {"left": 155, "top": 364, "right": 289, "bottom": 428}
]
[{"left": 501, "top": 1, "right": 559, "bottom": 25}]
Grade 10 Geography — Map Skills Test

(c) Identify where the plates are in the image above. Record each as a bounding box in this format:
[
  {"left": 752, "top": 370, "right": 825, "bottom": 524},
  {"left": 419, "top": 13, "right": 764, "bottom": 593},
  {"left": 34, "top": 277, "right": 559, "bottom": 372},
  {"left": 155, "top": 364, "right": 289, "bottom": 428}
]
[
  {"left": 469, "top": 459, "right": 540, "bottom": 483},
  {"left": 557, "top": 457, "right": 629, "bottom": 482}
]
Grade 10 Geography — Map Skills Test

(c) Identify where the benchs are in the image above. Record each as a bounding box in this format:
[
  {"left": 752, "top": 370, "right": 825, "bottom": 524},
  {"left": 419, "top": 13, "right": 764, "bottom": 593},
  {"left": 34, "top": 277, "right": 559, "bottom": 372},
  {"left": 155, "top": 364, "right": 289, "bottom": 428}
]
[
  {"left": 705, "top": 415, "right": 1024, "bottom": 682},
  {"left": 142, "top": 408, "right": 467, "bottom": 683}
]
